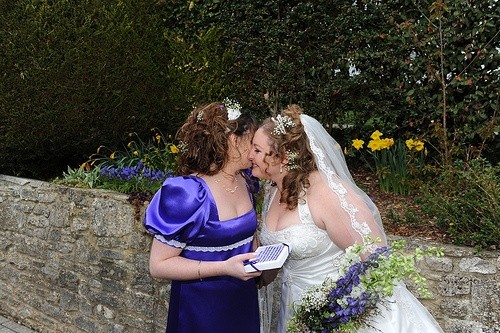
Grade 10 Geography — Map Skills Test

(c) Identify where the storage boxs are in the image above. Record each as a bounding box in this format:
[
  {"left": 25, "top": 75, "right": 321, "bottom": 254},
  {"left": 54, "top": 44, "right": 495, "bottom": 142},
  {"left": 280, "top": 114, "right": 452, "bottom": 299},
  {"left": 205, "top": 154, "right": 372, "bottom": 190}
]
[{"left": 244, "top": 242, "right": 292, "bottom": 272}]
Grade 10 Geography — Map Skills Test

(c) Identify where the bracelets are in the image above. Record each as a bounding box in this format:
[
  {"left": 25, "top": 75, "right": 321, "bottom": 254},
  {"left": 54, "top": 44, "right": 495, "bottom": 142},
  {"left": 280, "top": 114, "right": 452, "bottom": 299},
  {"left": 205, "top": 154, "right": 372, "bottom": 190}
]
[{"left": 198, "top": 260, "right": 202, "bottom": 281}]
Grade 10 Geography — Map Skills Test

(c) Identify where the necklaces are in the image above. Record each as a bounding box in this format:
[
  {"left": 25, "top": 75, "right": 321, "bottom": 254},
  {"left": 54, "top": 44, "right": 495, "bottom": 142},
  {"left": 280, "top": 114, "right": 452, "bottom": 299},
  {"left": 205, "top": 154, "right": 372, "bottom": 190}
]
[{"left": 213, "top": 173, "right": 239, "bottom": 193}]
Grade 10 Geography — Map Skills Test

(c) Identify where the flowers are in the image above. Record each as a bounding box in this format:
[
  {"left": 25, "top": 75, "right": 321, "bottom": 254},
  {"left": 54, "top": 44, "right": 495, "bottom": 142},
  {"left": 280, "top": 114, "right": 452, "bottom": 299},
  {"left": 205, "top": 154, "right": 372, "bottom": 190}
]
[
  {"left": 286, "top": 236, "right": 446, "bottom": 333},
  {"left": 270, "top": 115, "right": 296, "bottom": 137},
  {"left": 281, "top": 148, "right": 301, "bottom": 172},
  {"left": 224, "top": 97, "right": 241, "bottom": 120}
]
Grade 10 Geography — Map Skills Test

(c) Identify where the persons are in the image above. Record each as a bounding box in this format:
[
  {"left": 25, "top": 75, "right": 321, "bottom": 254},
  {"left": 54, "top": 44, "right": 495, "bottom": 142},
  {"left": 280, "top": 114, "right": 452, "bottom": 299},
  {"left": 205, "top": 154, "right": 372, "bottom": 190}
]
[
  {"left": 247, "top": 104, "right": 446, "bottom": 333},
  {"left": 144, "top": 97, "right": 279, "bottom": 333}
]
